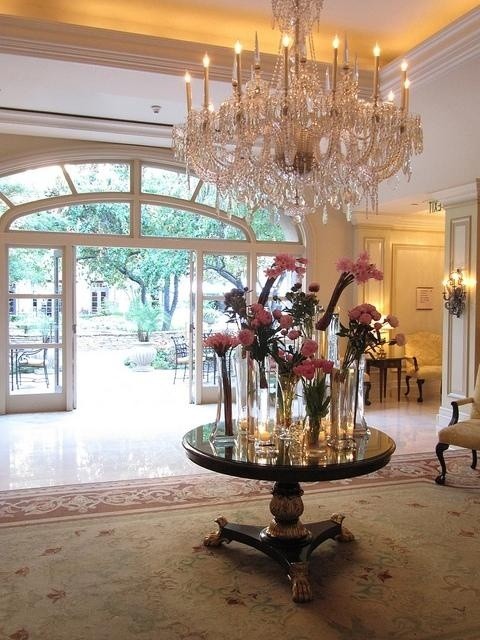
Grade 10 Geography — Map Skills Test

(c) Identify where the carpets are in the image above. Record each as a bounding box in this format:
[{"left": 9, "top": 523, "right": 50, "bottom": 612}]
[{"left": 0, "top": 448, "right": 480, "bottom": 640}]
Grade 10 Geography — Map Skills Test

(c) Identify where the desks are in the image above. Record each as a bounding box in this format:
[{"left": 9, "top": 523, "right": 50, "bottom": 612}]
[
  {"left": 8, "top": 341, "right": 63, "bottom": 391},
  {"left": 364, "top": 356, "right": 406, "bottom": 403},
  {"left": 182, "top": 417, "right": 396, "bottom": 604}
]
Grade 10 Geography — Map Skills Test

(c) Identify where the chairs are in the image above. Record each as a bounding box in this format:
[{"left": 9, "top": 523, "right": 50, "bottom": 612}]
[
  {"left": 435, "top": 362, "right": 480, "bottom": 484},
  {"left": 18, "top": 331, "right": 53, "bottom": 388},
  {"left": 405, "top": 331, "right": 443, "bottom": 402},
  {"left": 206, "top": 347, "right": 234, "bottom": 384},
  {"left": 363, "top": 372, "right": 372, "bottom": 406},
  {"left": 170, "top": 336, "right": 206, "bottom": 384}
]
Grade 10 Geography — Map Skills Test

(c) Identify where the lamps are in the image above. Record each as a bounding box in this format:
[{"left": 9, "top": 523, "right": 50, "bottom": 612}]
[
  {"left": 169, "top": 1, "right": 424, "bottom": 224},
  {"left": 442, "top": 269, "right": 466, "bottom": 318}
]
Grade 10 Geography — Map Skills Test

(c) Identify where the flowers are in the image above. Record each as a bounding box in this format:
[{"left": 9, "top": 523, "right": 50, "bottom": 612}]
[
  {"left": 315, "top": 249, "right": 383, "bottom": 332},
  {"left": 222, "top": 285, "right": 254, "bottom": 334},
  {"left": 237, "top": 302, "right": 292, "bottom": 389},
  {"left": 257, "top": 252, "right": 308, "bottom": 308},
  {"left": 352, "top": 314, "right": 407, "bottom": 428},
  {"left": 293, "top": 357, "right": 335, "bottom": 446},
  {"left": 335, "top": 303, "right": 381, "bottom": 384},
  {"left": 203, "top": 332, "right": 242, "bottom": 436}
]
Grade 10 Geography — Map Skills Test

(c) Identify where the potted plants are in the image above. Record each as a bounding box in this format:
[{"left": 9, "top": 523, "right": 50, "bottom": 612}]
[{"left": 124, "top": 295, "right": 172, "bottom": 371}]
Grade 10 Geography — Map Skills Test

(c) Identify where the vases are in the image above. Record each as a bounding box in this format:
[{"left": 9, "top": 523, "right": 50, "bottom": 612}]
[
  {"left": 232, "top": 348, "right": 248, "bottom": 435},
  {"left": 303, "top": 412, "right": 329, "bottom": 458},
  {"left": 254, "top": 363, "right": 280, "bottom": 454},
  {"left": 328, "top": 313, "right": 339, "bottom": 368},
  {"left": 348, "top": 353, "right": 370, "bottom": 435},
  {"left": 273, "top": 282, "right": 325, "bottom": 360},
  {"left": 245, "top": 351, "right": 254, "bottom": 442},
  {"left": 208, "top": 356, "right": 240, "bottom": 443},
  {"left": 274, "top": 374, "right": 297, "bottom": 441},
  {"left": 329, "top": 359, "right": 360, "bottom": 452}
]
